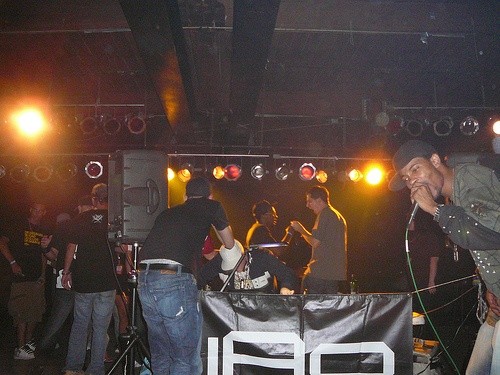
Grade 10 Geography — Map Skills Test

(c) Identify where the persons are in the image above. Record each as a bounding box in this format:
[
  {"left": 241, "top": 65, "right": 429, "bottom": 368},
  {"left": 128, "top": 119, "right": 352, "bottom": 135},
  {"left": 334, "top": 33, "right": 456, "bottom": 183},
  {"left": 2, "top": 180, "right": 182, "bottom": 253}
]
[
  {"left": 201, "top": 233, "right": 218, "bottom": 270},
  {"left": 199, "top": 239, "right": 297, "bottom": 295},
  {"left": 246, "top": 200, "right": 295, "bottom": 289},
  {"left": 138, "top": 177, "right": 235, "bottom": 375},
  {"left": 402, "top": 212, "right": 445, "bottom": 341},
  {"left": 0, "top": 196, "right": 114, "bottom": 375},
  {"left": 290, "top": 185, "right": 348, "bottom": 294},
  {"left": 60, "top": 183, "right": 120, "bottom": 375},
  {"left": 387, "top": 139, "right": 500, "bottom": 375}
]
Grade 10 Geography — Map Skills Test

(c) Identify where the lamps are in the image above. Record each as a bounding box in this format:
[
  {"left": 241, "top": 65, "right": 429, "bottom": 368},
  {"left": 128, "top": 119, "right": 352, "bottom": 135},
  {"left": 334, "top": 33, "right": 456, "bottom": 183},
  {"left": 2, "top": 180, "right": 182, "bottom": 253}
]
[
  {"left": 250, "top": 162, "right": 269, "bottom": 180},
  {"left": 274, "top": 163, "right": 289, "bottom": 181},
  {"left": 316, "top": 166, "right": 336, "bottom": 184},
  {"left": 177, "top": 163, "right": 193, "bottom": 182},
  {"left": 0, "top": 160, "right": 78, "bottom": 183},
  {"left": 298, "top": 160, "right": 318, "bottom": 181},
  {"left": 73, "top": 113, "right": 97, "bottom": 134},
  {"left": 344, "top": 166, "right": 362, "bottom": 182},
  {"left": 406, "top": 115, "right": 431, "bottom": 137},
  {"left": 125, "top": 112, "right": 146, "bottom": 134},
  {"left": 82, "top": 159, "right": 103, "bottom": 178},
  {"left": 458, "top": 114, "right": 480, "bottom": 136},
  {"left": 99, "top": 114, "right": 122, "bottom": 135},
  {"left": 203, "top": 161, "right": 243, "bottom": 183},
  {"left": 433, "top": 115, "right": 454, "bottom": 136}
]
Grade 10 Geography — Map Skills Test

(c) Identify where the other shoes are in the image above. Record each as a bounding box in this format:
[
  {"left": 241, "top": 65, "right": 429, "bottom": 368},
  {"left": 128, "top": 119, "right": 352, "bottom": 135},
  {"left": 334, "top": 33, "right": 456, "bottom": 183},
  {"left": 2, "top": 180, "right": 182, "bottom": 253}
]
[{"left": 66, "top": 369, "right": 84, "bottom": 375}]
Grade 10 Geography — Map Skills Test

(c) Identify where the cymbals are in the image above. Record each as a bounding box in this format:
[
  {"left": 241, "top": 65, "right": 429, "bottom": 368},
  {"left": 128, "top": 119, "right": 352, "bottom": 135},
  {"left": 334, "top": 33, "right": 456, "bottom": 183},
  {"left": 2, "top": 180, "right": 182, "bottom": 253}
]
[{"left": 109, "top": 219, "right": 130, "bottom": 225}]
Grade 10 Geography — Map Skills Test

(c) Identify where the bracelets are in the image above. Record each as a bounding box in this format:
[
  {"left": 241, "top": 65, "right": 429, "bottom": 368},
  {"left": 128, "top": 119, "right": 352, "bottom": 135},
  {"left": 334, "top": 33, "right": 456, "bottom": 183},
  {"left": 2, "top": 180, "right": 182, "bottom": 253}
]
[
  {"left": 433, "top": 204, "right": 445, "bottom": 223},
  {"left": 10, "top": 259, "right": 17, "bottom": 265},
  {"left": 287, "top": 231, "right": 294, "bottom": 238},
  {"left": 62, "top": 271, "right": 70, "bottom": 275}
]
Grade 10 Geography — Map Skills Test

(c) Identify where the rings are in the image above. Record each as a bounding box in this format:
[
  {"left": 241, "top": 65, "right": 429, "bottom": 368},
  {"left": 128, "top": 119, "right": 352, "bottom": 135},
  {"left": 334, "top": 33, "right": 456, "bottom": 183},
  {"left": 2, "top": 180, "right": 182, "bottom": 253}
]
[{"left": 46, "top": 246, "right": 48, "bottom": 248}]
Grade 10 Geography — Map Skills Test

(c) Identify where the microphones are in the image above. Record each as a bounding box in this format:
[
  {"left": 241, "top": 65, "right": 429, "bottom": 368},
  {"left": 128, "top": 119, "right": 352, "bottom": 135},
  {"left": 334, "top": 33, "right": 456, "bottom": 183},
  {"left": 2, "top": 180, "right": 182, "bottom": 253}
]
[
  {"left": 250, "top": 242, "right": 289, "bottom": 247},
  {"left": 408, "top": 202, "right": 419, "bottom": 224}
]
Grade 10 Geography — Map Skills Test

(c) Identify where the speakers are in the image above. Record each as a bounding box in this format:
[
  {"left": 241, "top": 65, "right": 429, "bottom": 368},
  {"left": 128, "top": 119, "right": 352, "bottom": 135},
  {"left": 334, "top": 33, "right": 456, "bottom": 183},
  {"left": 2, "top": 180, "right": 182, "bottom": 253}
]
[{"left": 107, "top": 149, "right": 168, "bottom": 244}]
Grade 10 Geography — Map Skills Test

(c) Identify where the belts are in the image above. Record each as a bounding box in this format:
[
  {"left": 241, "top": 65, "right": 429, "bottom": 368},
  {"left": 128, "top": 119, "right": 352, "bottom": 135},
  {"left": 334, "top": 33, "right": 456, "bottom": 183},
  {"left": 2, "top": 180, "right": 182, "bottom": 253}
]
[{"left": 138, "top": 262, "right": 193, "bottom": 274}]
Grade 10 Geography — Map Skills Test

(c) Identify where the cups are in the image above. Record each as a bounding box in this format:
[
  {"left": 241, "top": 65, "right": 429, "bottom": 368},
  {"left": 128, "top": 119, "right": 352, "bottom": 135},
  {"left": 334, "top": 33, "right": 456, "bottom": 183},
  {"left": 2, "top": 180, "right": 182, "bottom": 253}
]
[{"left": 350, "top": 282, "right": 359, "bottom": 294}]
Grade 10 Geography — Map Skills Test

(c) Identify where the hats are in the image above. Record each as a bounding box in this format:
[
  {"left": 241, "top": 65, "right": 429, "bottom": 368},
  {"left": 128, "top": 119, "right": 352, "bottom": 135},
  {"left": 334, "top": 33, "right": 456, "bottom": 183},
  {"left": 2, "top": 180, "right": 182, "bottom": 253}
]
[
  {"left": 388, "top": 140, "right": 433, "bottom": 192},
  {"left": 219, "top": 238, "right": 244, "bottom": 271}
]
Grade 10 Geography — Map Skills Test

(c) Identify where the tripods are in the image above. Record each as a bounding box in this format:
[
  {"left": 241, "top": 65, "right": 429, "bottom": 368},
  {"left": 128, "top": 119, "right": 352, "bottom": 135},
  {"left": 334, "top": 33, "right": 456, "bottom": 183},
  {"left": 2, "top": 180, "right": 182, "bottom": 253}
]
[{"left": 104, "top": 244, "right": 151, "bottom": 375}]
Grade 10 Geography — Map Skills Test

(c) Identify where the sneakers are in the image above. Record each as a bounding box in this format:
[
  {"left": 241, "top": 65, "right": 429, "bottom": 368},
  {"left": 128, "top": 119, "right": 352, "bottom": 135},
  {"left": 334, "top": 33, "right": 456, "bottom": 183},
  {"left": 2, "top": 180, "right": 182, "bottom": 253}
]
[
  {"left": 24, "top": 340, "right": 39, "bottom": 351},
  {"left": 13, "top": 346, "right": 36, "bottom": 360}
]
